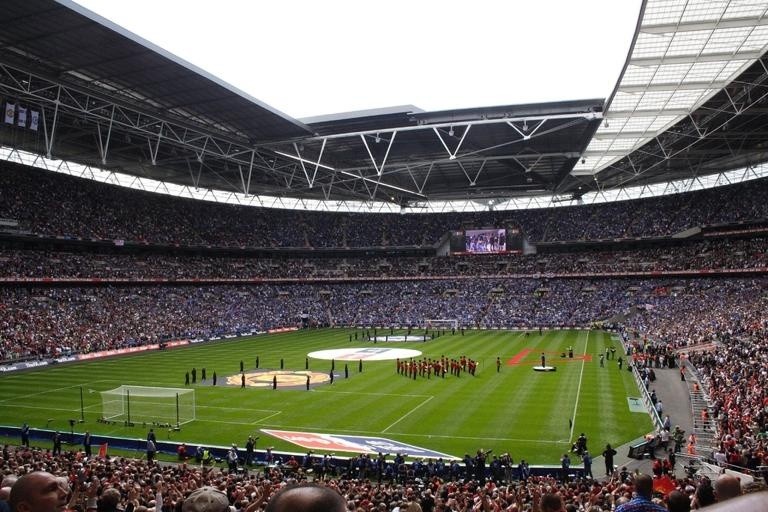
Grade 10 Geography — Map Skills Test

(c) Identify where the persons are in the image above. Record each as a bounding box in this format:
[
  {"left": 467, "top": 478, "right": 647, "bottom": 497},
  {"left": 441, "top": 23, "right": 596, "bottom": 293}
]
[
  {"left": 201, "top": 366, "right": 206, "bottom": 383},
  {"left": 330, "top": 369, "right": 334, "bottom": 384},
  {"left": 306, "top": 376, "right": 310, "bottom": 390},
  {"left": 0, "top": 157, "right": 768, "bottom": 379},
  {"left": 272, "top": 375, "right": 277, "bottom": 390},
  {"left": 213, "top": 370, "right": 217, "bottom": 386},
  {"left": 1, "top": 424, "right": 642, "bottom": 512},
  {"left": 191, "top": 368, "right": 197, "bottom": 384},
  {"left": 240, "top": 373, "right": 246, "bottom": 390},
  {"left": 642, "top": 380, "right": 768, "bottom": 511},
  {"left": 185, "top": 371, "right": 190, "bottom": 386}
]
